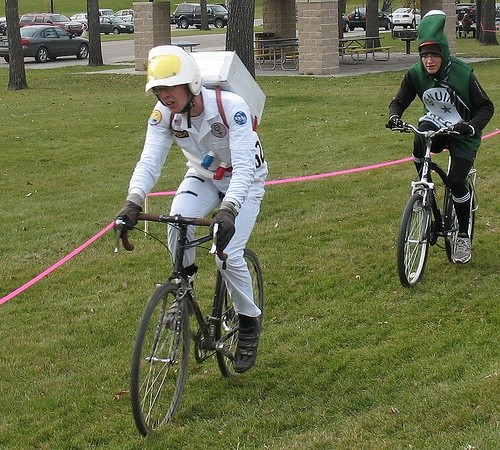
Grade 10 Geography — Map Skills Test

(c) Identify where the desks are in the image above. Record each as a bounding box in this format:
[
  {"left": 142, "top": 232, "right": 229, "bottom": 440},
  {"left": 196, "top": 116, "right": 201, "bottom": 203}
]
[
  {"left": 254, "top": 36, "right": 385, "bottom": 67},
  {"left": 171, "top": 43, "right": 200, "bottom": 52}
]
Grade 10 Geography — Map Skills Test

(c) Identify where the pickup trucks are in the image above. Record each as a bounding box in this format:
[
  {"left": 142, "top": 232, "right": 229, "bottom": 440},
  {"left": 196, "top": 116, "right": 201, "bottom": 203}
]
[{"left": 172, "top": 3, "right": 229, "bottom": 31}]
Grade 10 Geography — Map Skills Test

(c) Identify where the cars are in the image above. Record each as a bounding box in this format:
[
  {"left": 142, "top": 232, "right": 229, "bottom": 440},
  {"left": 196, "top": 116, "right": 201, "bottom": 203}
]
[
  {"left": 340, "top": 14, "right": 349, "bottom": 32},
  {"left": 97, "top": 16, "right": 134, "bottom": 34},
  {"left": 0, "top": 15, "right": 8, "bottom": 36},
  {"left": 493, "top": 2, "right": 500, "bottom": 20},
  {"left": 456, "top": 3, "right": 478, "bottom": 23},
  {"left": 69, "top": 8, "right": 177, "bottom": 31},
  {"left": 347, "top": 8, "right": 391, "bottom": 32},
  {"left": 0, "top": 26, "right": 90, "bottom": 64},
  {"left": 389, "top": 8, "right": 421, "bottom": 29}
]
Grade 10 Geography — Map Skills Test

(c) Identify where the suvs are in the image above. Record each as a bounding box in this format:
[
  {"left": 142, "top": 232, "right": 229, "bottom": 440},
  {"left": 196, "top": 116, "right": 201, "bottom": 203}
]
[{"left": 19, "top": 13, "right": 83, "bottom": 37}]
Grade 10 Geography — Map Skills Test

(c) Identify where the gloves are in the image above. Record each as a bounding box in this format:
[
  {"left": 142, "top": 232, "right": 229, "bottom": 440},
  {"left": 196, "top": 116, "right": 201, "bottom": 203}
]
[
  {"left": 389, "top": 116, "right": 403, "bottom": 133},
  {"left": 454, "top": 123, "right": 472, "bottom": 137},
  {"left": 114, "top": 201, "right": 140, "bottom": 238},
  {"left": 210, "top": 209, "right": 235, "bottom": 251}
]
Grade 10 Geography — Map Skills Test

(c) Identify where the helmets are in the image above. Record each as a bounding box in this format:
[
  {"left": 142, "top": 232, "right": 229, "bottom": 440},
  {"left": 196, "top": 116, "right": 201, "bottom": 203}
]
[{"left": 145, "top": 45, "right": 203, "bottom": 96}]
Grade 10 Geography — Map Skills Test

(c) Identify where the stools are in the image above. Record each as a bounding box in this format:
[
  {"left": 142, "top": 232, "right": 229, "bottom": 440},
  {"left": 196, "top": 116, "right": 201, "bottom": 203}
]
[{"left": 457, "top": 31, "right": 468, "bottom": 39}]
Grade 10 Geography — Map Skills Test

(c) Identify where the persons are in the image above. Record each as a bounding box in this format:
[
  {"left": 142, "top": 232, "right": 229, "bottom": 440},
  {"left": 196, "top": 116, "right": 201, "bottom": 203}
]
[
  {"left": 456, "top": 14, "right": 475, "bottom": 37},
  {"left": 387, "top": 9, "right": 495, "bottom": 264},
  {"left": 114, "top": 45, "right": 269, "bottom": 373}
]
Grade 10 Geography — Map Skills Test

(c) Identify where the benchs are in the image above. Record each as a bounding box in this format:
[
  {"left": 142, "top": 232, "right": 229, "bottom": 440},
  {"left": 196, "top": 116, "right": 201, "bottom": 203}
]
[{"left": 254, "top": 46, "right": 394, "bottom": 72}]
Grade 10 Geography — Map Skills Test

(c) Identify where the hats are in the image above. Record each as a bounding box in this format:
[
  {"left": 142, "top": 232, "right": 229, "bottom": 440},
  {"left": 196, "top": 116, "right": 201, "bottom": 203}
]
[{"left": 419, "top": 44, "right": 442, "bottom": 56}]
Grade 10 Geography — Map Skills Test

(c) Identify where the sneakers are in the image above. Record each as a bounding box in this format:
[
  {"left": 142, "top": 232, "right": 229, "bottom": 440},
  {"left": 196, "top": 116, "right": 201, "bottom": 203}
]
[
  {"left": 164, "top": 297, "right": 191, "bottom": 326},
  {"left": 235, "top": 319, "right": 259, "bottom": 373},
  {"left": 453, "top": 233, "right": 472, "bottom": 263},
  {"left": 412, "top": 200, "right": 422, "bottom": 212}
]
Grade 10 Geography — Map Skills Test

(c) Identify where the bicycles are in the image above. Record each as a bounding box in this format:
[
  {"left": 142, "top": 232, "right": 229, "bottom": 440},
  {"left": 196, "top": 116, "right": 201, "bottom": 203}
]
[
  {"left": 114, "top": 211, "right": 264, "bottom": 437},
  {"left": 384, "top": 121, "right": 478, "bottom": 290}
]
